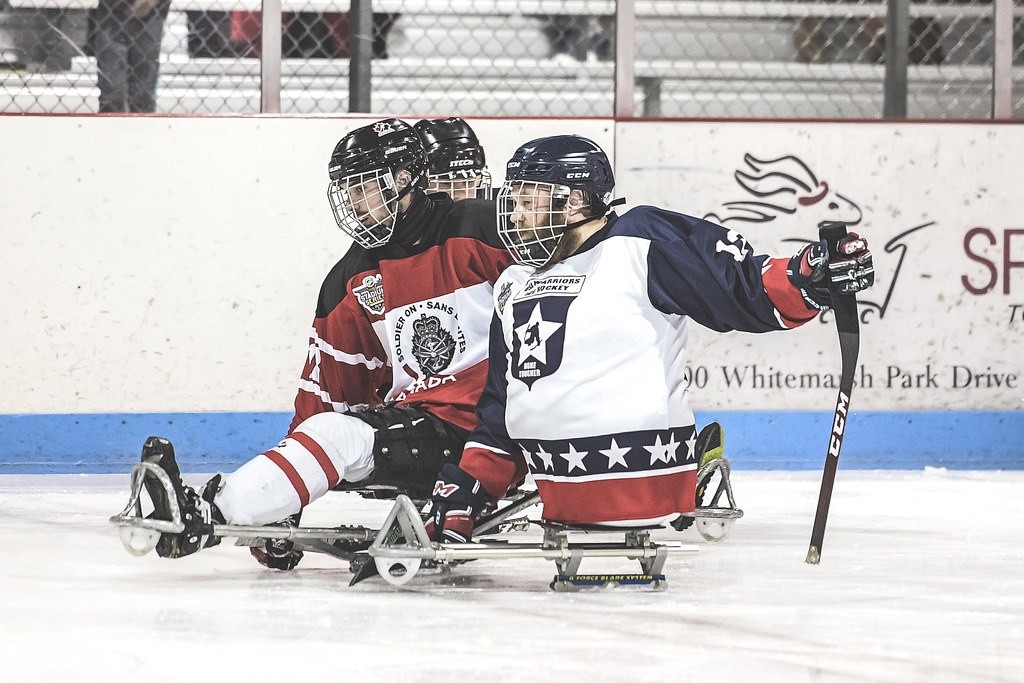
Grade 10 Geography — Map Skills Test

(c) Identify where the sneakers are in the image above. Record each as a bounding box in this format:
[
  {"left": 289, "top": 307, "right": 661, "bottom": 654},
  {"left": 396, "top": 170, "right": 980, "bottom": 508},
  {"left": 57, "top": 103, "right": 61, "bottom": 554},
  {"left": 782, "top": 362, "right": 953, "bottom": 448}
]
[
  {"left": 139, "top": 436, "right": 225, "bottom": 559},
  {"left": 654, "top": 421, "right": 725, "bottom": 532}
]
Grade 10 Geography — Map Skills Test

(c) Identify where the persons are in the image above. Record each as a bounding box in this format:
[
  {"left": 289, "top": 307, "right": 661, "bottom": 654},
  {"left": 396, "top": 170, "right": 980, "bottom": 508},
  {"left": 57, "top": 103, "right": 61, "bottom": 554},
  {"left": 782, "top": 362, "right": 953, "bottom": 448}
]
[
  {"left": 417, "top": 136, "right": 876, "bottom": 548},
  {"left": 141, "top": 117, "right": 515, "bottom": 569},
  {"left": 94, "top": 0, "right": 171, "bottom": 113}
]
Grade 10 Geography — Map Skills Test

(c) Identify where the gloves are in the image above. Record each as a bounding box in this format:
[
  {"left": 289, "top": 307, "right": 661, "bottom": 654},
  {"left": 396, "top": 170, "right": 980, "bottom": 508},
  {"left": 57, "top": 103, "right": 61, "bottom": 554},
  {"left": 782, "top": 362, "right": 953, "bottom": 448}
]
[
  {"left": 251, "top": 512, "right": 304, "bottom": 571},
  {"left": 786, "top": 232, "right": 875, "bottom": 311},
  {"left": 412, "top": 462, "right": 498, "bottom": 570}
]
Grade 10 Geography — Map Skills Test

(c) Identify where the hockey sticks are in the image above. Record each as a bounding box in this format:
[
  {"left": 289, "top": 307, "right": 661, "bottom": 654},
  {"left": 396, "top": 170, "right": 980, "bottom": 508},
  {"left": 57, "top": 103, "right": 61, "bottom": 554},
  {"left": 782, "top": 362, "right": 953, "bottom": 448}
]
[{"left": 804, "top": 219, "right": 862, "bottom": 566}]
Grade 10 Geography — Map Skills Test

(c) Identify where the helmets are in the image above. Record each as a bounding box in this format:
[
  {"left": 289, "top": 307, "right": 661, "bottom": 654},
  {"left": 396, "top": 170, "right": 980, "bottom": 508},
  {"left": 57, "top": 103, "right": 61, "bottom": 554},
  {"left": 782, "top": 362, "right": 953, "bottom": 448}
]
[
  {"left": 496, "top": 135, "right": 615, "bottom": 268},
  {"left": 413, "top": 117, "right": 493, "bottom": 200},
  {"left": 327, "top": 118, "right": 429, "bottom": 250}
]
